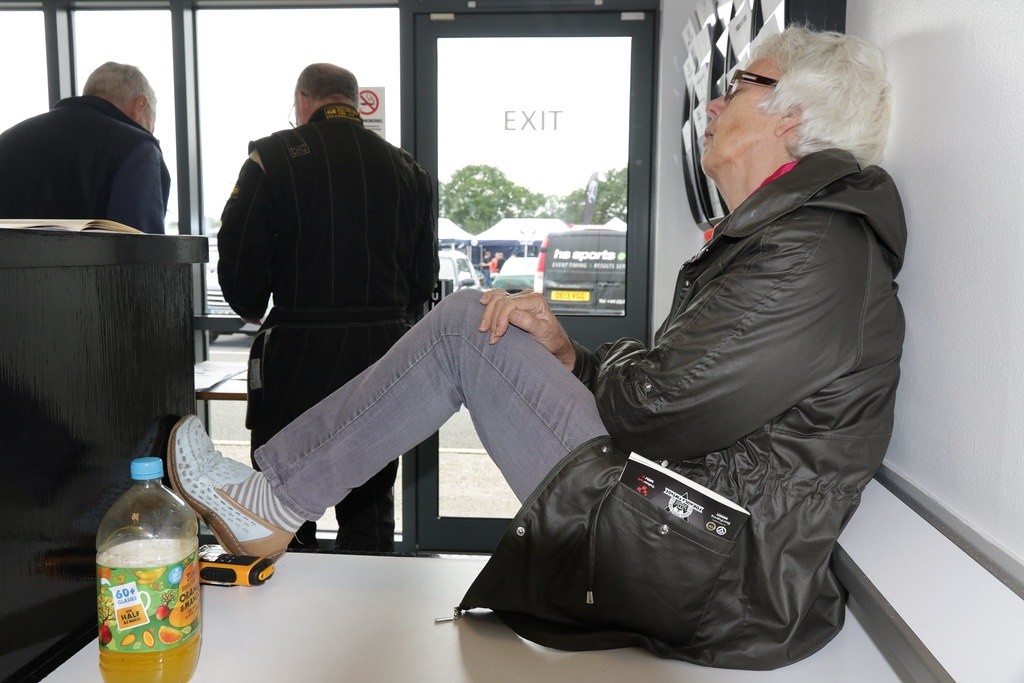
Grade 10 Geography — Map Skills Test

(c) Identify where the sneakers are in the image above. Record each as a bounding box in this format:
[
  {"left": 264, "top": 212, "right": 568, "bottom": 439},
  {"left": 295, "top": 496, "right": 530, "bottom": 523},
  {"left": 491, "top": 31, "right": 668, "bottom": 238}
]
[{"left": 168, "top": 413, "right": 295, "bottom": 566}]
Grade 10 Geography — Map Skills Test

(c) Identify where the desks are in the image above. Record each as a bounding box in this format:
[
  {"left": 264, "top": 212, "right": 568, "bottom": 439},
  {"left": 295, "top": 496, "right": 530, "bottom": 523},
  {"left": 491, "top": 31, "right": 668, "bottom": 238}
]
[{"left": 35, "top": 548, "right": 908, "bottom": 683}]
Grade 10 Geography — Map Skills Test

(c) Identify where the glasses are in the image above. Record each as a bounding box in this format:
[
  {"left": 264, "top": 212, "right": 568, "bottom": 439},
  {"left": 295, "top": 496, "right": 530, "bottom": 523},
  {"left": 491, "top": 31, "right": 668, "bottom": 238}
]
[
  {"left": 724, "top": 68, "right": 779, "bottom": 101},
  {"left": 287, "top": 94, "right": 299, "bottom": 129}
]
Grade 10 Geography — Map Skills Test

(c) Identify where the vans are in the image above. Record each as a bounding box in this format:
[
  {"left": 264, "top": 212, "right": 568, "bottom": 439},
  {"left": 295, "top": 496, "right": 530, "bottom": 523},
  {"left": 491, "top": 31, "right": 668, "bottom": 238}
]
[{"left": 438, "top": 216, "right": 627, "bottom": 316}]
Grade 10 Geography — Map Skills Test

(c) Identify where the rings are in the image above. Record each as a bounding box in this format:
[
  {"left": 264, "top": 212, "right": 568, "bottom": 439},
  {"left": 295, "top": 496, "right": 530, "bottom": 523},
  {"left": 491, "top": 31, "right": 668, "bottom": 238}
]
[{"left": 506, "top": 293, "right": 510, "bottom": 297}]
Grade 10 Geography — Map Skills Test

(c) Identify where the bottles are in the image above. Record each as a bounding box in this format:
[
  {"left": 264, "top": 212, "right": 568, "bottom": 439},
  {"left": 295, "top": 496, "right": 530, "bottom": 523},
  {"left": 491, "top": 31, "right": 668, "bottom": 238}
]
[{"left": 95, "top": 456, "right": 202, "bottom": 683}]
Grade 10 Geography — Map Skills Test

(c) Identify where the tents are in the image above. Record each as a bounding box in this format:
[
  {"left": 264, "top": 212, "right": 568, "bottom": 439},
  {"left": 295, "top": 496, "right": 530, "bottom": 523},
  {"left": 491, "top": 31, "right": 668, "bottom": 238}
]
[
  {"left": 478, "top": 219, "right": 570, "bottom": 257},
  {"left": 438, "top": 218, "right": 474, "bottom": 261}
]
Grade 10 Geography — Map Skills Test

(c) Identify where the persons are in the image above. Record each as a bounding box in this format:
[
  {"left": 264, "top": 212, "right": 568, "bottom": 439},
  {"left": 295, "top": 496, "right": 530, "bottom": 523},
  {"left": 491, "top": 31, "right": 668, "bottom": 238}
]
[
  {"left": 480, "top": 251, "right": 493, "bottom": 289},
  {"left": 167, "top": 23, "right": 907, "bottom": 671},
  {"left": 490, "top": 252, "right": 504, "bottom": 284},
  {"left": 0, "top": 61, "right": 170, "bottom": 234},
  {"left": 219, "top": 63, "right": 439, "bottom": 555}
]
